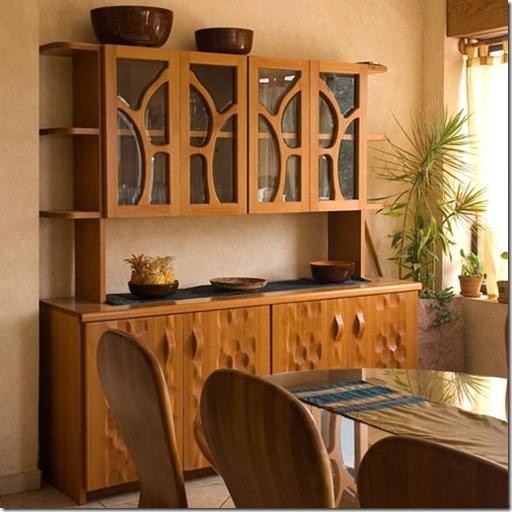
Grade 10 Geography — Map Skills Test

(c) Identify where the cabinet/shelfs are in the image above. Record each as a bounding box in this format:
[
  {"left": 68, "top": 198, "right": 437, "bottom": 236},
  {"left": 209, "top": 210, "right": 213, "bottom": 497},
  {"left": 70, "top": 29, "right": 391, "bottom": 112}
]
[{"left": 40, "top": 38, "right": 423, "bottom": 508}]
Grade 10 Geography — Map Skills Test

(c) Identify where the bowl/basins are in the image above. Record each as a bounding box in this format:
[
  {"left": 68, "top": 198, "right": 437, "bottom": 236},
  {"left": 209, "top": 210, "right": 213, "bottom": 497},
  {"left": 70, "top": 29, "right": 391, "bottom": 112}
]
[
  {"left": 309, "top": 259, "right": 356, "bottom": 283},
  {"left": 127, "top": 280, "right": 178, "bottom": 298},
  {"left": 194, "top": 27, "right": 253, "bottom": 55},
  {"left": 90, "top": 5, "right": 175, "bottom": 50}
]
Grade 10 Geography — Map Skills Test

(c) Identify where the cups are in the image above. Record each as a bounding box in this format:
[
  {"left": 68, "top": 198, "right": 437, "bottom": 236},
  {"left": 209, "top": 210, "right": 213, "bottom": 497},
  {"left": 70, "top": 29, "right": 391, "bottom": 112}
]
[{"left": 118, "top": 183, "right": 168, "bottom": 204}]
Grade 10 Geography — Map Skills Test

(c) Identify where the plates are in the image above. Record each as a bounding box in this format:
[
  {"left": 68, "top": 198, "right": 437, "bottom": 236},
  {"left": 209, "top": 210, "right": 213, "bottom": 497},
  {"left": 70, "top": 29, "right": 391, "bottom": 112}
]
[{"left": 210, "top": 276, "right": 266, "bottom": 292}]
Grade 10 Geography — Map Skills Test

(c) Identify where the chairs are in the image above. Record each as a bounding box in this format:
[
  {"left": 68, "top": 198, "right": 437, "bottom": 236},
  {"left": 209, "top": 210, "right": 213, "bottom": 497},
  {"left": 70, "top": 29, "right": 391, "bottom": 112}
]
[
  {"left": 95, "top": 327, "right": 187, "bottom": 509},
  {"left": 357, "top": 437, "right": 508, "bottom": 508},
  {"left": 199, "top": 368, "right": 335, "bottom": 508}
]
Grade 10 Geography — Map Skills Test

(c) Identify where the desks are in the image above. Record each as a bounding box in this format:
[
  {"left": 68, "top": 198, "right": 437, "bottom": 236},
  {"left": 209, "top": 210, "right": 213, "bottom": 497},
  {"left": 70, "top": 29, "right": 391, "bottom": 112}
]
[{"left": 261, "top": 367, "right": 508, "bottom": 508}]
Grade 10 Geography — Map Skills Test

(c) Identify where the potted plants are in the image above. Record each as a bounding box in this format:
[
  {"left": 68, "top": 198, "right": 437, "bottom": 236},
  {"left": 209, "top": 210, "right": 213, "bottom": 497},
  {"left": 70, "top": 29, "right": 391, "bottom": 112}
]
[{"left": 458, "top": 249, "right": 482, "bottom": 298}]
